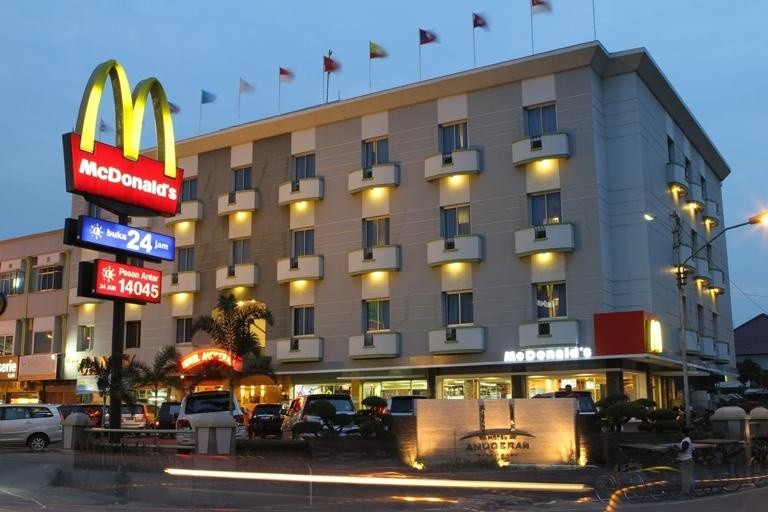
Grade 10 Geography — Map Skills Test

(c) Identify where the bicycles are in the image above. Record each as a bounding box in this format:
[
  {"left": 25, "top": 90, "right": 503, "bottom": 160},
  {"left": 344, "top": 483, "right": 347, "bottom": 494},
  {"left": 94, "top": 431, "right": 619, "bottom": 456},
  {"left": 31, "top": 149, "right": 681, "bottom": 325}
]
[{"left": 592, "top": 441, "right": 767, "bottom": 507}]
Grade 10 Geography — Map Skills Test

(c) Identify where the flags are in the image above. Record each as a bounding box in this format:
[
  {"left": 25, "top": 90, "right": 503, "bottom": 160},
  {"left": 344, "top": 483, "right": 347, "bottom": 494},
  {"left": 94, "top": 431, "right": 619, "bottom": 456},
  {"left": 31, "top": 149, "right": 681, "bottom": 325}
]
[
  {"left": 323, "top": 56, "right": 342, "bottom": 72},
  {"left": 371, "top": 42, "right": 390, "bottom": 58},
  {"left": 201, "top": 89, "right": 218, "bottom": 104},
  {"left": 168, "top": 101, "right": 181, "bottom": 113},
  {"left": 473, "top": 12, "right": 487, "bottom": 30},
  {"left": 100, "top": 119, "right": 115, "bottom": 133},
  {"left": 420, "top": 29, "right": 437, "bottom": 45},
  {"left": 240, "top": 77, "right": 255, "bottom": 94},
  {"left": 279, "top": 67, "right": 296, "bottom": 83},
  {"left": 532, "top": 0, "right": 552, "bottom": 14}
]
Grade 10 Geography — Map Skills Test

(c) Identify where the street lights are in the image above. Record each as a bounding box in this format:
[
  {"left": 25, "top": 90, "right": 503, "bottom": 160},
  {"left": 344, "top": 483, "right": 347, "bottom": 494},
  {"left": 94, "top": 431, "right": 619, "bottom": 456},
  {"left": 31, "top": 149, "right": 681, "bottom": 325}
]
[{"left": 644, "top": 209, "right": 768, "bottom": 429}]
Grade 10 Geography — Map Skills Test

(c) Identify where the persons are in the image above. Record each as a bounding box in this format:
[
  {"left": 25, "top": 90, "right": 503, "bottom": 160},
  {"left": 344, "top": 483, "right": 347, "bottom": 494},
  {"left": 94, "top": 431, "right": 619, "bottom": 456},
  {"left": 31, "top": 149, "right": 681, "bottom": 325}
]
[
  {"left": 672, "top": 425, "right": 695, "bottom": 493},
  {"left": 565, "top": 384, "right": 572, "bottom": 392}
]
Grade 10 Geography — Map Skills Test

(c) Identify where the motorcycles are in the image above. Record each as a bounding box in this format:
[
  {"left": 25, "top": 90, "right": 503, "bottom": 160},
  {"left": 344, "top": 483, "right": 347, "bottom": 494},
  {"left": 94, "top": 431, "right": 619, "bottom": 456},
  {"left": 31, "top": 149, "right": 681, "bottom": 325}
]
[{"left": 676, "top": 401, "right": 713, "bottom": 431}]
